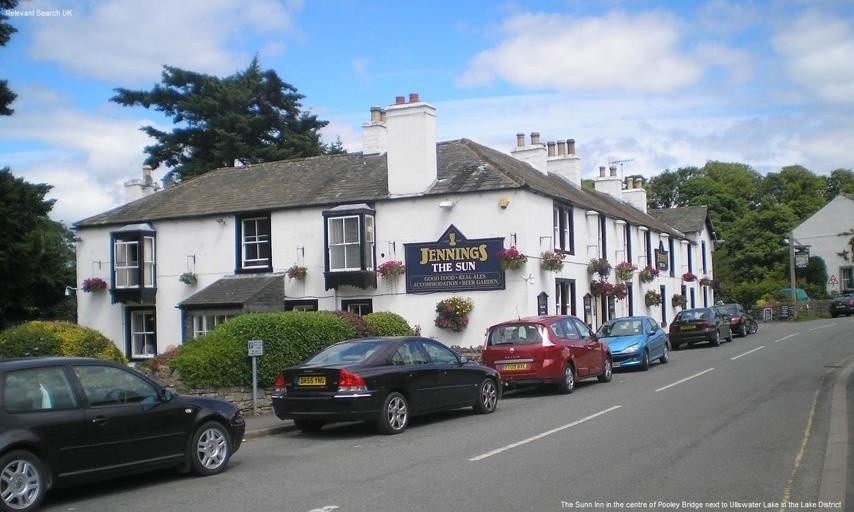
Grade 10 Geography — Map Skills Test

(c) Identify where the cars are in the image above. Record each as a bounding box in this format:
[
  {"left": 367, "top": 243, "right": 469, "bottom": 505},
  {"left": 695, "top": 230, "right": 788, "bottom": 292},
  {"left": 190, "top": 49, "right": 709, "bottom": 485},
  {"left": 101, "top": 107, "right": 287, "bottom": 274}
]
[
  {"left": 713, "top": 303, "right": 759, "bottom": 337},
  {"left": 0, "top": 354, "right": 247, "bottom": 511},
  {"left": 269, "top": 335, "right": 504, "bottom": 434},
  {"left": 829, "top": 288, "right": 854, "bottom": 318},
  {"left": 669, "top": 306, "right": 733, "bottom": 349},
  {"left": 480, "top": 314, "right": 612, "bottom": 396},
  {"left": 592, "top": 316, "right": 670, "bottom": 372}
]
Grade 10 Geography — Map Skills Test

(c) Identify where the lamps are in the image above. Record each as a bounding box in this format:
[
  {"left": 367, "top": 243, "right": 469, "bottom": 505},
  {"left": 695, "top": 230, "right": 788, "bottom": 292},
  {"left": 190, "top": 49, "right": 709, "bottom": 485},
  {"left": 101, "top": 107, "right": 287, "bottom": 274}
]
[
  {"left": 70, "top": 231, "right": 81, "bottom": 249},
  {"left": 64, "top": 285, "right": 78, "bottom": 298},
  {"left": 437, "top": 199, "right": 458, "bottom": 209},
  {"left": 214, "top": 217, "right": 227, "bottom": 225},
  {"left": 584, "top": 210, "right": 691, "bottom": 245},
  {"left": 521, "top": 271, "right": 535, "bottom": 285}
]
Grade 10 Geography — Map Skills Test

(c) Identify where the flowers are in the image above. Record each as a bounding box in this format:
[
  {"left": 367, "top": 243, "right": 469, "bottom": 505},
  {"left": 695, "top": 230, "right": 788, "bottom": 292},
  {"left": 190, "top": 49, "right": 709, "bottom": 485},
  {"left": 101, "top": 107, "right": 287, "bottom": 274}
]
[
  {"left": 375, "top": 260, "right": 405, "bottom": 279},
  {"left": 681, "top": 271, "right": 698, "bottom": 283},
  {"left": 495, "top": 245, "right": 527, "bottom": 270},
  {"left": 587, "top": 257, "right": 688, "bottom": 308},
  {"left": 286, "top": 265, "right": 307, "bottom": 280},
  {"left": 539, "top": 248, "right": 566, "bottom": 272},
  {"left": 81, "top": 276, "right": 106, "bottom": 293},
  {"left": 699, "top": 278, "right": 715, "bottom": 289},
  {"left": 177, "top": 272, "right": 196, "bottom": 286},
  {"left": 433, "top": 296, "right": 475, "bottom": 330}
]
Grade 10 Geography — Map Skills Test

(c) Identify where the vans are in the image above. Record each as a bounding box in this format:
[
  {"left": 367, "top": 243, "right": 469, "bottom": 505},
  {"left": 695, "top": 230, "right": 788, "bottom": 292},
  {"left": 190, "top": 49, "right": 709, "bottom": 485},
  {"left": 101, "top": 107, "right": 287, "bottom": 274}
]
[{"left": 781, "top": 288, "right": 808, "bottom": 302}]
